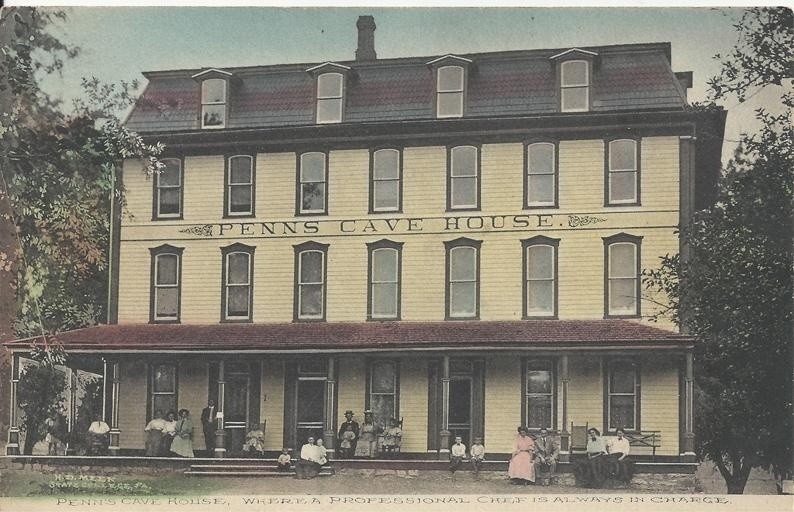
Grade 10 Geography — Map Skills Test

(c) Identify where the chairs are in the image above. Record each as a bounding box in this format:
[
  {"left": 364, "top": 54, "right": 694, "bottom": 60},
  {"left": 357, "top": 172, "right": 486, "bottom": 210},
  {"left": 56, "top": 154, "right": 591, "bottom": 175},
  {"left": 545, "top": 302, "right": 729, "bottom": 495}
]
[
  {"left": 568, "top": 420, "right": 590, "bottom": 461},
  {"left": 382, "top": 416, "right": 403, "bottom": 457}
]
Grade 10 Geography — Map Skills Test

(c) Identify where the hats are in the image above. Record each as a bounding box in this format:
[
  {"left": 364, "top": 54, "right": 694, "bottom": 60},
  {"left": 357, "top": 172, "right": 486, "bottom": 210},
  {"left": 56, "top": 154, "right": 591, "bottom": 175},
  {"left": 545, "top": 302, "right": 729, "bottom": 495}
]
[
  {"left": 364, "top": 409, "right": 372, "bottom": 415},
  {"left": 588, "top": 428, "right": 600, "bottom": 436},
  {"left": 344, "top": 411, "right": 354, "bottom": 416},
  {"left": 517, "top": 426, "right": 529, "bottom": 432},
  {"left": 177, "top": 408, "right": 189, "bottom": 416}
]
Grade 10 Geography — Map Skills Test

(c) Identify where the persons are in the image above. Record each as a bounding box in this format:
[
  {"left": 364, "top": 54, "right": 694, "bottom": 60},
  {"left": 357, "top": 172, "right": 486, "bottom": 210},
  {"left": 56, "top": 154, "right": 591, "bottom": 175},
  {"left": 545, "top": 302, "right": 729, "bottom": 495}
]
[
  {"left": 310, "top": 437, "right": 328, "bottom": 465},
  {"left": 605, "top": 427, "right": 631, "bottom": 488},
  {"left": 143, "top": 407, "right": 166, "bottom": 457},
  {"left": 353, "top": 409, "right": 375, "bottom": 459},
  {"left": 293, "top": 435, "right": 318, "bottom": 479},
  {"left": 160, "top": 409, "right": 179, "bottom": 455},
  {"left": 585, "top": 425, "right": 609, "bottom": 480},
  {"left": 88, "top": 410, "right": 112, "bottom": 456},
  {"left": 507, "top": 425, "right": 538, "bottom": 488},
  {"left": 469, "top": 434, "right": 485, "bottom": 478},
  {"left": 337, "top": 409, "right": 361, "bottom": 459},
  {"left": 201, "top": 397, "right": 220, "bottom": 459},
  {"left": 42, "top": 408, "right": 61, "bottom": 455},
  {"left": 446, "top": 434, "right": 467, "bottom": 476},
  {"left": 379, "top": 417, "right": 404, "bottom": 459},
  {"left": 533, "top": 425, "right": 561, "bottom": 487},
  {"left": 277, "top": 445, "right": 292, "bottom": 473},
  {"left": 170, "top": 407, "right": 196, "bottom": 458}
]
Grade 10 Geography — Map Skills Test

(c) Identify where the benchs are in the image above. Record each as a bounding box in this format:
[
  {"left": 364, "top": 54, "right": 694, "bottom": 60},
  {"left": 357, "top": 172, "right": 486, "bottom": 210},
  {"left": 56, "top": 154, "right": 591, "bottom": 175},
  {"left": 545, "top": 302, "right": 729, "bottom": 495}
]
[{"left": 594, "top": 430, "right": 660, "bottom": 463}]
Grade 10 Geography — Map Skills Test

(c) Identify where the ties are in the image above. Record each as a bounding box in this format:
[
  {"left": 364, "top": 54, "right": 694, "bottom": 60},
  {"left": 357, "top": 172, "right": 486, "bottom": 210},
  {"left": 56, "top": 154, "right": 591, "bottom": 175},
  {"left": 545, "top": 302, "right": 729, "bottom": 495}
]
[{"left": 542, "top": 438, "right": 547, "bottom": 447}]
